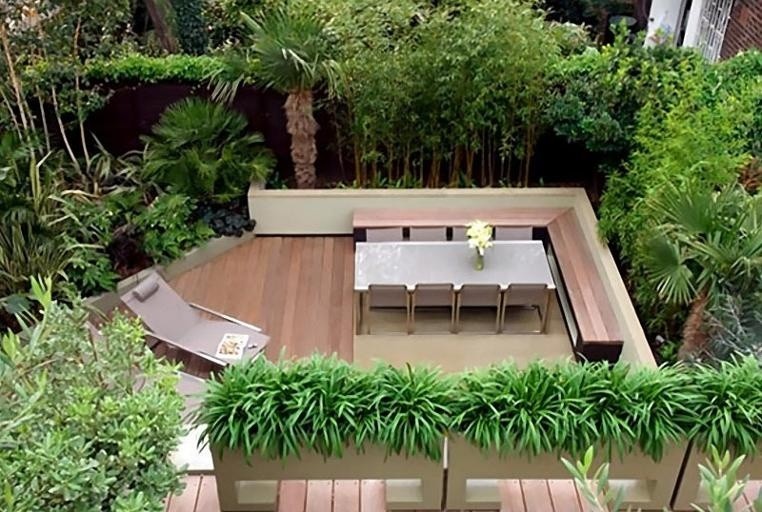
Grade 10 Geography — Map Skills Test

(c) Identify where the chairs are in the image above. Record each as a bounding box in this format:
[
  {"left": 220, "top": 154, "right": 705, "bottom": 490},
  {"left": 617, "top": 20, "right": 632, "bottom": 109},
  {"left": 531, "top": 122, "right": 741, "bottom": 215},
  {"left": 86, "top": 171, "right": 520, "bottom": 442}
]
[
  {"left": 354, "top": 225, "right": 557, "bottom": 334},
  {"left": 121, "top": 266, "right": 273, "bottom": 369}
]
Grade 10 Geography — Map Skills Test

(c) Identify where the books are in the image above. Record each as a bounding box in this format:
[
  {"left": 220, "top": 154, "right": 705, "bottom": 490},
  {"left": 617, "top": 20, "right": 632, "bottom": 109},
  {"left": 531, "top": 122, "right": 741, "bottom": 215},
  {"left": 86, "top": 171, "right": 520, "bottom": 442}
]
[{"left": 215, "top": 332, "right": 250, "bottom": 362}]
